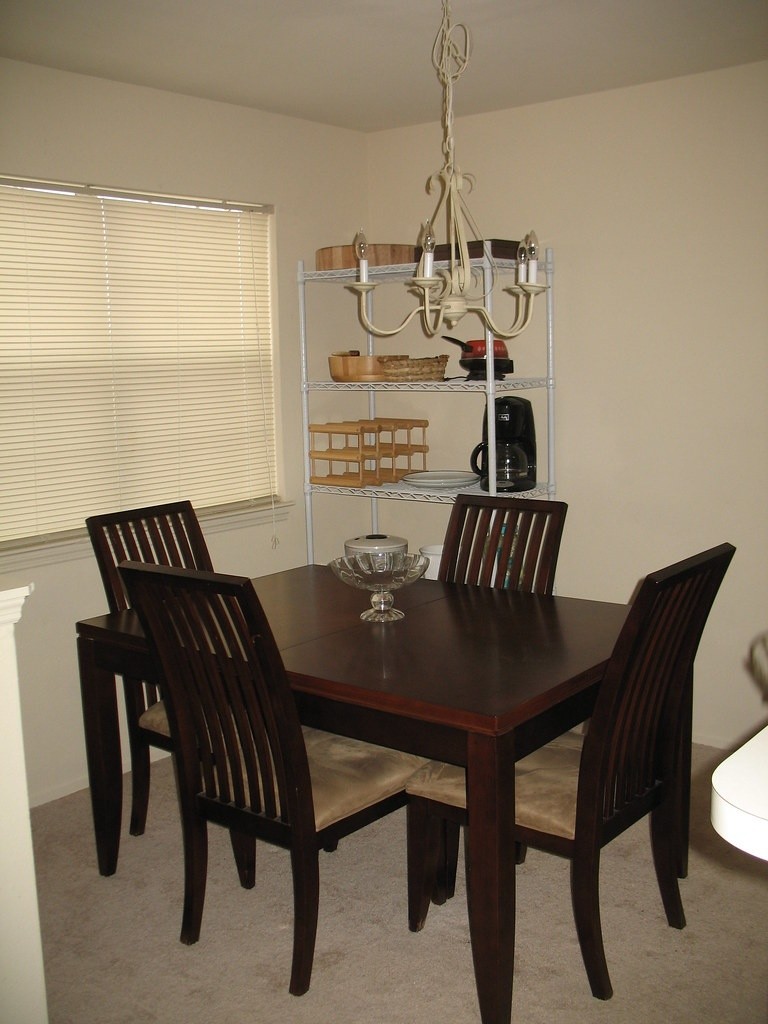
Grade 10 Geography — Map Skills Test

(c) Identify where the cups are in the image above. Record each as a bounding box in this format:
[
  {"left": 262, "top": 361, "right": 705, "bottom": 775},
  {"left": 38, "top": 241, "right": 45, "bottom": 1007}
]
[{"left": 420, "top": 544, "right": 443, "bottom": 579}]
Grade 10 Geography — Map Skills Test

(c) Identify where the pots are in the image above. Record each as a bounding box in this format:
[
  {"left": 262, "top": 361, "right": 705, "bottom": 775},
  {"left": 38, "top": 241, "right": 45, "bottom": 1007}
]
[{"left": 442, "top": 334, "right": 509, "bottom": 358}]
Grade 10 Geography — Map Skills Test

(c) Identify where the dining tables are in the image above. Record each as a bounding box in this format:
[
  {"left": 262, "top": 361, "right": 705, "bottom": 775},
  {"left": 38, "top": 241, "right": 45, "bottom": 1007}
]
[{"left": 75, "top": 563, "right": 631, "bottom": 1024}]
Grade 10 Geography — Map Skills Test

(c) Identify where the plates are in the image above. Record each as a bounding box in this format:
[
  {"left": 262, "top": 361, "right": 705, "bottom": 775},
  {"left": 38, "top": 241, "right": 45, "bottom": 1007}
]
[{"left": 402, "top": 471, "right": 481, "bottom": 486}]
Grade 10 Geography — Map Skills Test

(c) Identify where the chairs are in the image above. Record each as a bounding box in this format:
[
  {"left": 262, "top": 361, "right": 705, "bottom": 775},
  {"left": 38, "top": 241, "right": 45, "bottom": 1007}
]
[{"left": 84, "top": 495, "right": 737, "bottom": 990}]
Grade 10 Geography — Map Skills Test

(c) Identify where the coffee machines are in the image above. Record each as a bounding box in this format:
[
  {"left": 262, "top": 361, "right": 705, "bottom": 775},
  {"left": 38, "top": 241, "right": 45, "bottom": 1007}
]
[{"left": 473, "top": 397, "right": 539, "bottom": 488}]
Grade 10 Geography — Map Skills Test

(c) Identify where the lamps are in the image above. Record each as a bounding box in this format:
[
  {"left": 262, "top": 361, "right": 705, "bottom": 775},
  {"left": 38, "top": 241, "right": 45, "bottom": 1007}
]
[{"left": 344, "top": 1, "right": 549, "bottom": 340}]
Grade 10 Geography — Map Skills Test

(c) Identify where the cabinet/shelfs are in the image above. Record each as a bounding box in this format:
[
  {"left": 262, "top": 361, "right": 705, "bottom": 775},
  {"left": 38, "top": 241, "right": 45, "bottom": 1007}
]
[{"left": 297, "top": 249, "right": 559, "bottom": 564}]
